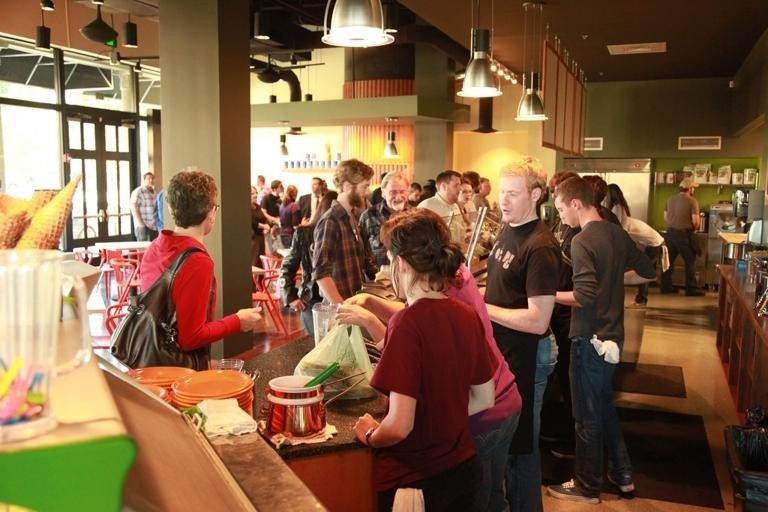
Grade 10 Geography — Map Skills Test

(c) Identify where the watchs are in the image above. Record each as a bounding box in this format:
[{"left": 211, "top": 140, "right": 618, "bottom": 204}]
[{"left": 366, "top": 427, "right": 375, "bottom": 448}]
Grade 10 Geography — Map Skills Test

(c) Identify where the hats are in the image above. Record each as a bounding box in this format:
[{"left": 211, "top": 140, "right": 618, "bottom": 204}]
[{"left": 680, "top": 179, "right": 699, "bottom": 189}]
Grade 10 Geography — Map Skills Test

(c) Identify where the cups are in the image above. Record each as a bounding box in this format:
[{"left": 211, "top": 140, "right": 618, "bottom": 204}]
[
  {"left": 217, "top": 358, "right": 244, "bottom": 373},
  {"left": 666, "top": 173, "right": 673, "bottom": 183},
  {"left": 283, "top": 153, "right": 340, "bottom": 169}
]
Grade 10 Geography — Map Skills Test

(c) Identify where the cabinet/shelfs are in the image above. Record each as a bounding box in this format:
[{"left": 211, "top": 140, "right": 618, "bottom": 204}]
[{"left": 715, "top": 261, "right": 768, "bottom": 415}]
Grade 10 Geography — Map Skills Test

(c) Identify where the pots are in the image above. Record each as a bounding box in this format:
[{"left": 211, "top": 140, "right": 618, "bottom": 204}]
[
  {"left": 259, "top": 372, "right": 367, "bottom": 437},
  {"left": 725, "top": 242, "right": 751, "bottom": 262},
  {"left": 751, "top": 254, "right": 768, "bottom": 316}
]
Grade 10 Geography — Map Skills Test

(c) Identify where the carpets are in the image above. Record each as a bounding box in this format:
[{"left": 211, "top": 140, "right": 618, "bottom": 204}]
[
  {"left": 540, "top": 406, "right": 728, "bottom": 511},
  {"left": 611, "top": 361, "right": 689, "bottom": 399}
]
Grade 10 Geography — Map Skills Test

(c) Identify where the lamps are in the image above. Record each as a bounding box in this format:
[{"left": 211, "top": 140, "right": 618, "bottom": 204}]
[
  {"left": 513, "top": 1, "right": 553, "bottom": 122},
  {"left": 121, "top": 12, "right": 140, "bottom": 51},
  {"left": 320, "top": 0, "right": 397, "bottom": 50},
  {"left": 382, "top": 116, "right": 401, "bottom": 161},
  {"left": 455, "top": 1, "right": 504, "bottom": 101},
  {"left": 35, "top": 3, "right": 54, "bottom": 53}
]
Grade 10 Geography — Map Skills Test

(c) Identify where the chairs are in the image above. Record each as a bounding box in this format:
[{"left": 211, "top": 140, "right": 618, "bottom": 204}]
[{"left": 71, "top": 236, "right": 306, "bottom": 340}]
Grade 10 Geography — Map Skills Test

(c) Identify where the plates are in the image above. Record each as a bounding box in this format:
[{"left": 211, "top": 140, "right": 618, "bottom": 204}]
[{"left": 128, "top": 365, "right": 254, "bottom": 418}]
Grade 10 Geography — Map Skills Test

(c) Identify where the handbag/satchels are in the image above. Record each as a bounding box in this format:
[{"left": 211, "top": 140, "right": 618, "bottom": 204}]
[{"left": 111, "top": 277, "right": 210, "bottom": 371}]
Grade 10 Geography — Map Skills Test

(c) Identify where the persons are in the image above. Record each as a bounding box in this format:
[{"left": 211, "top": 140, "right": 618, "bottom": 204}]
[
  {"left": 482, "top": 160, "right": 667, "bottom": 503},
  {"left": 131, "top": 173, "right": 159, "bottom": 241},
  {"left": 660, "top": 177, "right": 706, "bottom": 296},
  {"left": 353, "top": 207, "right": 499, "bottom": 512},
  {"left": 252, "top": 159, "right": 497, "bottom": 334},
  {"left": 334, "top": 214, "right": 522, "bottom": 512},
  {"left": 154, "top": 189, "right": 164, "bottom": 233},
  {"left": 137, "top": 172, "right": 262, "bottom": 367}
]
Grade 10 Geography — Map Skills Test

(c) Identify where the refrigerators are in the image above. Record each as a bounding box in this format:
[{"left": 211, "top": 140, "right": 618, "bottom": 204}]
[{"left": 563, "top": 157, "right": 652, "bottom": 226}]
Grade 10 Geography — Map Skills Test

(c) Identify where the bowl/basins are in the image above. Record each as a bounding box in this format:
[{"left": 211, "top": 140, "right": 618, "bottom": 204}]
[{"left": 718, "top": 212, "right": 734, "bottom": 222}]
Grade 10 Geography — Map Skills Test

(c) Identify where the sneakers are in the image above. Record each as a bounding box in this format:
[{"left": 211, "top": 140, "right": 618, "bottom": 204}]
[
  {"left": 661, "top": 286, "right": 679, "bottom": 294},
  {"left": 606, "top": 473, "right": 638, "bottom": 497},
  {"left": 547, "top": 479, "right": 600, "bottom": 504},
  {"left": 686, "top": 288, "right": 706, "bottom": 296}
]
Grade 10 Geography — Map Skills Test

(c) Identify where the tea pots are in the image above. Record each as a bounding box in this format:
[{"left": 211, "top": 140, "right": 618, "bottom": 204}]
[
  {"left": 311, "top": 302, "right": 341, "bottom": 348},
  {"left": 1, "top": 247, "right": 95, "bottom": 447}
]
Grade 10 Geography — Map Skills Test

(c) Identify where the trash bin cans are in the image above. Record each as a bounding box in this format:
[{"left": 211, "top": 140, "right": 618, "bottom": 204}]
[{"left": 724, "top": 424, "right": 768, "bottom": 511}]
